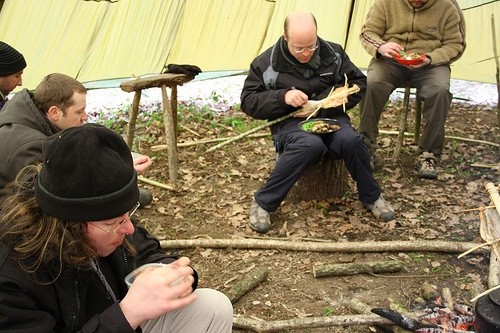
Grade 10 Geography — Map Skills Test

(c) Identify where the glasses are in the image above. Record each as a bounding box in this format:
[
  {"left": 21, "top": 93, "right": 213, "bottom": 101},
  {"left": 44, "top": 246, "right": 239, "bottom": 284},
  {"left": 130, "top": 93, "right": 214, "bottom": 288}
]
[
  {"left": 291, "top": 43, "right": 319, "bottom": 54},
  {"left": 87, "top": 202, "right": 140, "bottom": 237}
]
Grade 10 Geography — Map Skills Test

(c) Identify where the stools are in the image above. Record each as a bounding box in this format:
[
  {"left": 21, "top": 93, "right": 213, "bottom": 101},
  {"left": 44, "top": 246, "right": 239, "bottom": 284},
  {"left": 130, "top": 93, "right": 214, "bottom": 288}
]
[
  {"left": 393, "top": 80, "right": 422, "bottom": 164},
  {"left": 119, "top": 73, "right": 195, "bottom": 181}
]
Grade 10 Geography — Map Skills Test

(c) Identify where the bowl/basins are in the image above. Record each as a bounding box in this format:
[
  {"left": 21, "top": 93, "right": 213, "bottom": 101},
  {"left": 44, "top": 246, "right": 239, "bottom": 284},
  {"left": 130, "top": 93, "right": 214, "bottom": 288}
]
[
  {"left": 394, "top": 52, "right": 425, "bottom": 65},
  {"left": 125, "top": 261, "right": 186, "bottom": 291}
]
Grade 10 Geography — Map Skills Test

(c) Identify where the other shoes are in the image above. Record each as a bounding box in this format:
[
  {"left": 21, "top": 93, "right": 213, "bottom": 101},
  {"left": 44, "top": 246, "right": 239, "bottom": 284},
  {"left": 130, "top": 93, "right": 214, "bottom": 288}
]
[
  {"left": 249, "top": 197, "right": 271, "bottom": 233},
  {"left": 362, "top": 193, "right": 396, "bottom": 222},
  {"left": 139, "top": 188, "right": 153, "bottom": 210}
]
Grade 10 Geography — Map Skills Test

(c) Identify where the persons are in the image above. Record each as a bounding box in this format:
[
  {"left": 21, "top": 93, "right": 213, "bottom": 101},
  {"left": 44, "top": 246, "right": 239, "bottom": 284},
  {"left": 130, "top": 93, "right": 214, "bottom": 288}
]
[
  {"left": 360, "top": 0, "right": 466, "bottom": 179},
  {"left": 240, "top": 9, "right": 394, "bottom": 233},
  {"left": 0, "top": 41, "right": 27, "bottom": 112},
  {"left": 0, "top": 73, "right": 153, "bottom": 208},
  {"left": 0, "top": 122, "right": 233, "bottom": 333}
]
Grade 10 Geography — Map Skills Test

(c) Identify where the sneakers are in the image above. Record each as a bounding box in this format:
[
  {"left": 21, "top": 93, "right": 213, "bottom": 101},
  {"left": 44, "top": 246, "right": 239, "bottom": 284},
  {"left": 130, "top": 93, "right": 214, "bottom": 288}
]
[{"left": 419, "top": 152, "right": 438, "bottom": 179}]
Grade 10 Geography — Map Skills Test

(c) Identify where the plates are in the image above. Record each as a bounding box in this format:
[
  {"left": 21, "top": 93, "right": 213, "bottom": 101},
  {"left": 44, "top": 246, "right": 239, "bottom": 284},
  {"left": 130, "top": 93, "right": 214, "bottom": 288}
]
[{"left": 298, "top": 117, "right": 343, "bottom": 133}]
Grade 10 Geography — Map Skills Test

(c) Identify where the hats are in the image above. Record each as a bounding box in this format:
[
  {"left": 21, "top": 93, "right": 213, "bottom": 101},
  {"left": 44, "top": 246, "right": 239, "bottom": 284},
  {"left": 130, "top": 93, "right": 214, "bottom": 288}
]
[
  {"left": 0, "top": 40, "right": 27, "bottom": 77},
  {"left": 34, "top": 123, "right": 140, "bottom": 221}
]
